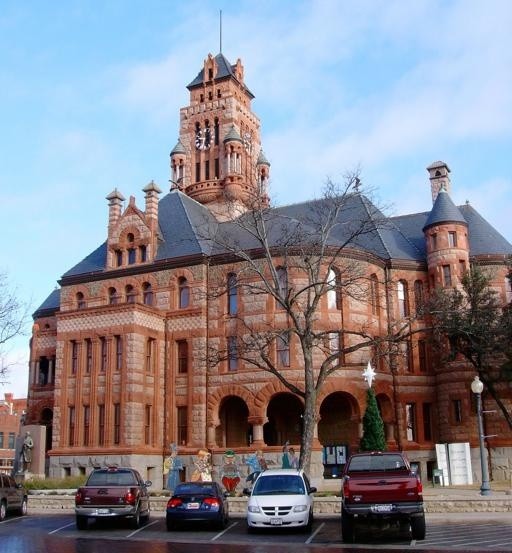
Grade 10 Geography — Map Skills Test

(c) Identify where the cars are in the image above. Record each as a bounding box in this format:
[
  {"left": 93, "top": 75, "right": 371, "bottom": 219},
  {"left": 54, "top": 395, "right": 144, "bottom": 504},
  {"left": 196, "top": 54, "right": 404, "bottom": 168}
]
[
  {"left": 166, "top": 482, "right": 230, "bottom": 529},
  {"left": 245, "top": 468, "right": 316, "bottom": 531},
  {"left": 0, "top": 472, "right": 28, "bottom": 519}
]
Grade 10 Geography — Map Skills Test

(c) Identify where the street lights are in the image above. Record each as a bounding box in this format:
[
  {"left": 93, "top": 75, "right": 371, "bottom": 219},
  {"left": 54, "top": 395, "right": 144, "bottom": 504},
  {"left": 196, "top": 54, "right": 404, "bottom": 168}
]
[{"left": 470, "top": 376, "right": 492, "bottom": 495}]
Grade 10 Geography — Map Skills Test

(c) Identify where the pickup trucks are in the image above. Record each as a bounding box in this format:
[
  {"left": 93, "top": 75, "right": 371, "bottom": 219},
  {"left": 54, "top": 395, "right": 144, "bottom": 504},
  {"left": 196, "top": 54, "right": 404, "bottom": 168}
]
[
  {"left": 341, "top": 451, "right": 427, "bottom": 540},
  {"left": 75, "top": 467, "right": 151, "bottom": 526}
]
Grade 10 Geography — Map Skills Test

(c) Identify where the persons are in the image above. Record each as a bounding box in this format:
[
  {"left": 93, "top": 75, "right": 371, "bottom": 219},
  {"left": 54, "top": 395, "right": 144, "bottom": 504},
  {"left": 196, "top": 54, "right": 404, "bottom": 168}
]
[{"left": 17, "top": 431, "right": 33, "bottom": 472}]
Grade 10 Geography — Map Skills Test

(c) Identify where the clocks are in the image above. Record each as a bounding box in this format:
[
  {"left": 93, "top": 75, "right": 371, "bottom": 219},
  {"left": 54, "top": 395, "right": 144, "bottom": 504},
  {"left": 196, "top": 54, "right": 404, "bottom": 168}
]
[{"left": 194, "top": 127, "right": 214, "bottom": 150}]
